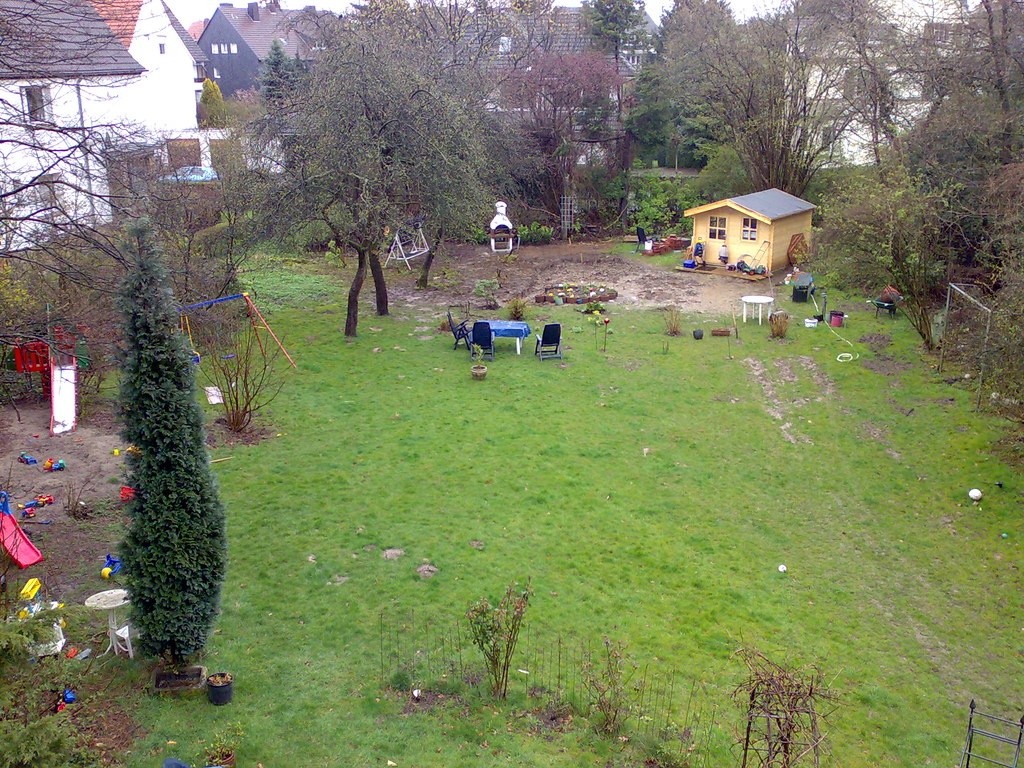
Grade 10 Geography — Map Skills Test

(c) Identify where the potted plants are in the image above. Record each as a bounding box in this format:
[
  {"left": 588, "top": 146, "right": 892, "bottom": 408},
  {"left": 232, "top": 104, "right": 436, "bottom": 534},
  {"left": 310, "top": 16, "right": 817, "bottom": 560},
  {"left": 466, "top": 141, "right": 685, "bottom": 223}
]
[
  {"left": 204, "top": 721, "right": 247, "bottom": 768},
  {"left": 535, "top": 283, "right": 617, "bottom": 304},
  {"left": 206, "top": 672, "right": 233, "bottom": 706}
]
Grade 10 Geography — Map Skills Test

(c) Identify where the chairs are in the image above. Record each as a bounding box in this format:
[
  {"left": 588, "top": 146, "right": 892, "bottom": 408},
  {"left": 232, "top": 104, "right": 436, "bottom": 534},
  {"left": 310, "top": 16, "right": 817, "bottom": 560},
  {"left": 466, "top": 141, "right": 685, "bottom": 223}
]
[
  {"left": 535, "top": 323, "right": 562, "bottom": 362},
  {"left": 114, "top": 617, "right": 143, "bottom": 658},
  {"left": 447, "top": 310, "right": 473, "bottom": 350},
  {"left": 469, "top": 321, "right": 495, "bottom": 358},
  {"left": 636, "top": 226, "right": 648, "bottom": 254}
]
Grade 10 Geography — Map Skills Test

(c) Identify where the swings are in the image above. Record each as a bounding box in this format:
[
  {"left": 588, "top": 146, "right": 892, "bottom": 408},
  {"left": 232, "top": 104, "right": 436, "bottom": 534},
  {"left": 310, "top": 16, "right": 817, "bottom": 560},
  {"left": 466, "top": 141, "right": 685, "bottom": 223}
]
[
  {"left": 170, "top": 315, "right": 202, "bottom": 367},
  {"left": 206, "top": 301, "right": 239, "bottom": 360}
]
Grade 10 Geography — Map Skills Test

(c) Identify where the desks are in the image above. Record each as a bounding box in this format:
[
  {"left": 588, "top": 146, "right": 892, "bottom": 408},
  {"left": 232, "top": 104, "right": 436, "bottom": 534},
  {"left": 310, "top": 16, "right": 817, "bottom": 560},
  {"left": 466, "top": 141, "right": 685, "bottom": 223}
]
[
  {"left": 476, "top": 320, "right": 532, "bottom": 355},
  {"left": 85, "top": 589, "right": 131, "bottom": 659},
  {"left": 741, "top": 295, "right": 774, "bottom": 326}
]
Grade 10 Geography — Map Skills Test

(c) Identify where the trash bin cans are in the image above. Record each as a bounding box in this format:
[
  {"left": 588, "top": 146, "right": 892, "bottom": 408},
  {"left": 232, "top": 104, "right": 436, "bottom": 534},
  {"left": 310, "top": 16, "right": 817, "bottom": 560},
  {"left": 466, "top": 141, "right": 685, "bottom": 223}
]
[{"left": 830, "top": 310, "right": 844, "bottom": 327}]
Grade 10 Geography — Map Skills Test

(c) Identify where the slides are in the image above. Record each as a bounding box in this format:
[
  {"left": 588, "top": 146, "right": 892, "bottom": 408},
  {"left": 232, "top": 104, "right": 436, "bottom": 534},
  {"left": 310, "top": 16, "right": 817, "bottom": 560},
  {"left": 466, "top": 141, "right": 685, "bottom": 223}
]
[{"left": 48, "top": 356, "right": 79, "bottom": 437}]
